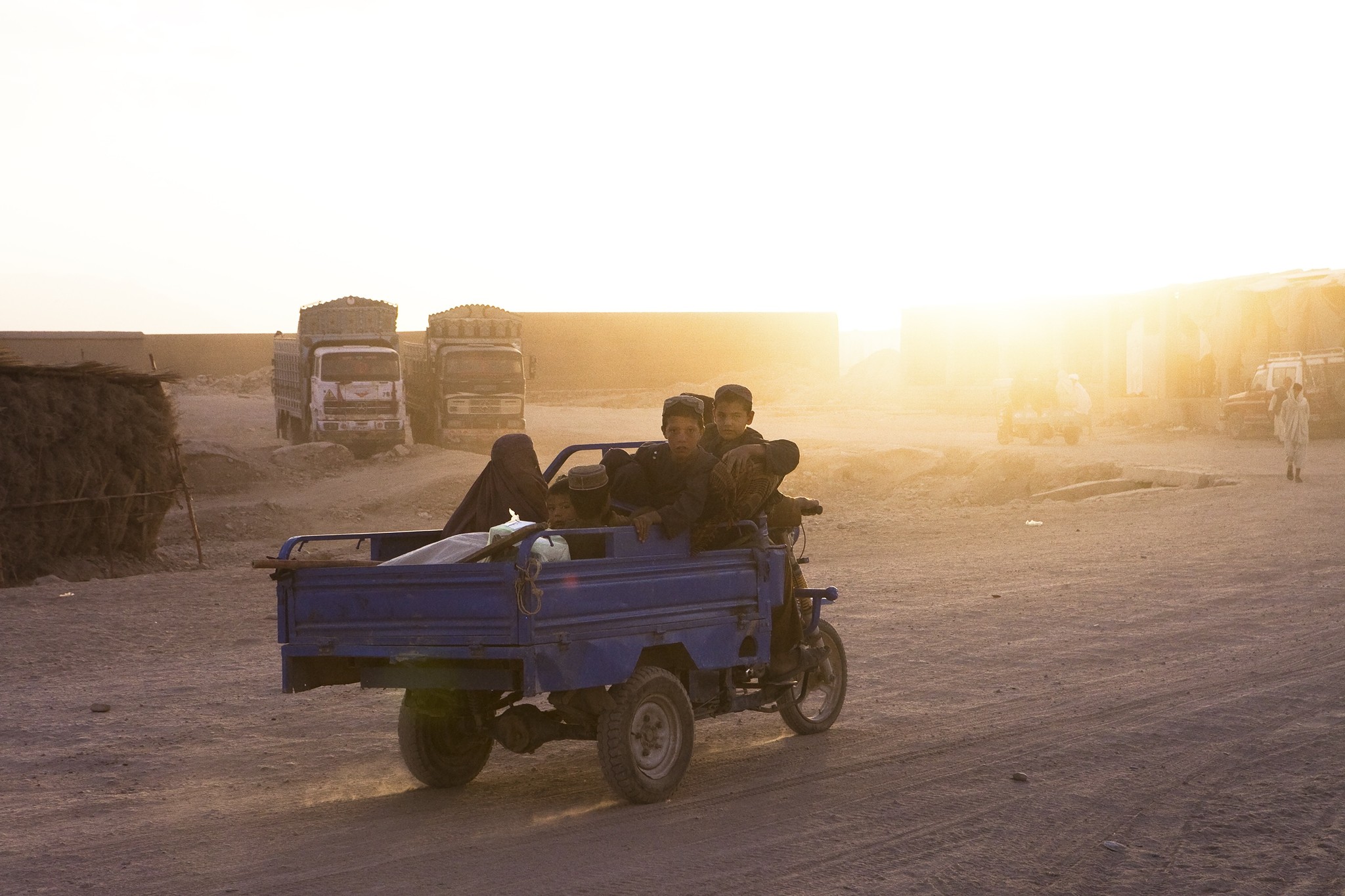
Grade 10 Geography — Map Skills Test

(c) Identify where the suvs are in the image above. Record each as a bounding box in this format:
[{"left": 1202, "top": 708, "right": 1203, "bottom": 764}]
[{"left": 1217, "top": 349, "right": 1345, "bottom": 439}]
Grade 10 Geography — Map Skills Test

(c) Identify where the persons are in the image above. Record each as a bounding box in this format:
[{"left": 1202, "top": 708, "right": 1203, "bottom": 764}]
[
  {"left": 699, "top": 385, "right": 800, "bottom": 550},
  {"left": 1268, "top": 377, "right": 1292, "bottom": 446},
  {"left": 681, "top": 392, "right": 831, "bottom": 680},
  {"left": 548, "top": 476, "right": 575, "bottom": 528},
  {"left": 1281, "top": 383, "right": 1310, "bottom": 483},
  {"left": 598, "top": 395, "right": 709, "bottom": 544},
  {"left": 563, "top": 464, "right": 630, "bottom": 560}
]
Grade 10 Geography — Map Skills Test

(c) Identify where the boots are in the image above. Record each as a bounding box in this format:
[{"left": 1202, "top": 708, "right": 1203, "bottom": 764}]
[
  {"left": 1294, "top": 468, "right": 1302, "bottom": 483},
  {"left": 1287, "top": 465, "right": 1294, "bottom": 480}
]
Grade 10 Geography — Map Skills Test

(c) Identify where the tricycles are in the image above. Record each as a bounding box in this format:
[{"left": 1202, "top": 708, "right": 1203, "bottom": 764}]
[{"left": 996, "top": 382, "right": 1084, "bottom": 446}]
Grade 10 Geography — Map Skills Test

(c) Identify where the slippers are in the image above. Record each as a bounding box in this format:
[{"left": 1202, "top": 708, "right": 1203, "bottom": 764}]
[{"left": 776, "top": 646, "right": 831, "bottom": 682}]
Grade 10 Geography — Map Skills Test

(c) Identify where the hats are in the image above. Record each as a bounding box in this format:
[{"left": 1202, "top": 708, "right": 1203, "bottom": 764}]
[
  {"left": 662, "top": 396, "right": 704, "bottom": 419},
  {"left": 713, "top": 384, "right": 753, "bottom": 404},
  {"left": 568, "top": 464, "right": 609, "bottom": 490}
]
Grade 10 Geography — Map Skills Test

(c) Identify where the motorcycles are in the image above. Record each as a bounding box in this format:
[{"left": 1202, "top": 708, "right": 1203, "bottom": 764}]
[{"left": 268, "top": 442, "right": 849, "bottom": 805}]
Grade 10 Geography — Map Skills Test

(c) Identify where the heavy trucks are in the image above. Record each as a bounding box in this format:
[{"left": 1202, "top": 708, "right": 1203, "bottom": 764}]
[
  {"left": 402, "top": 304, "right": 537, "bottom": 447},
  {"left": 269, "top": 296, "right": 408, "bottom": 451}
]
